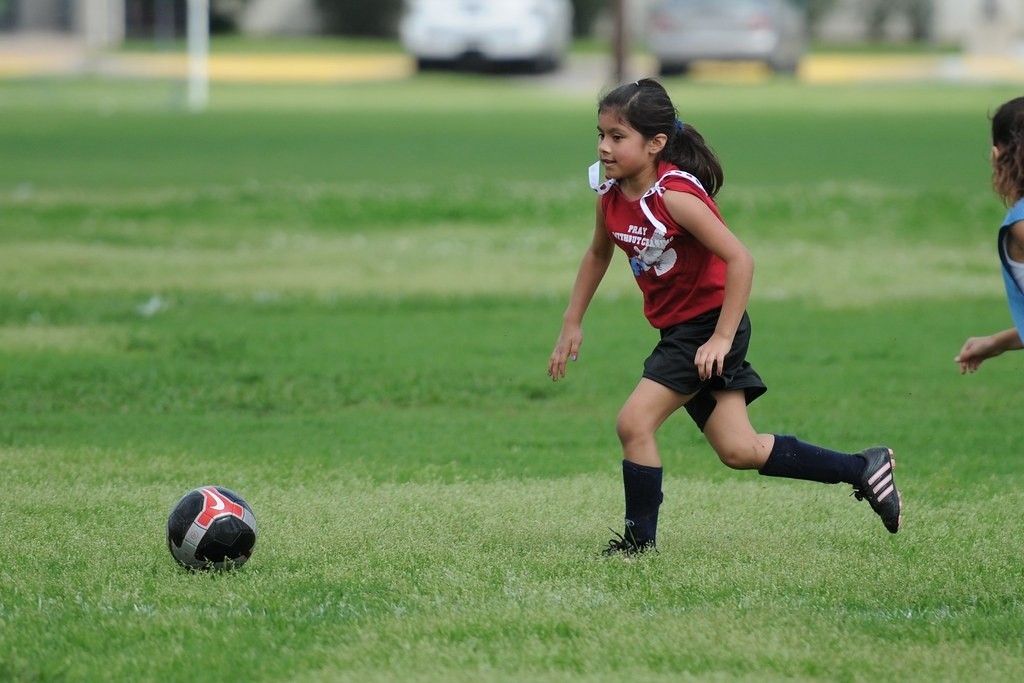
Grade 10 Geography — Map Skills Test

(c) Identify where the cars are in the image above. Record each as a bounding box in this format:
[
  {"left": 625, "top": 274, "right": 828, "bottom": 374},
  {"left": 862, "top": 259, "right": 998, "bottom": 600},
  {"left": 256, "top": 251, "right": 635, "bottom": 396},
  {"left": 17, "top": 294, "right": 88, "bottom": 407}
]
[
  {"left": 401, "top": 0, "right": 573, "bottom": 74},
  {"left": 644, "top": 0, "right": 804, "bottom": 76}
]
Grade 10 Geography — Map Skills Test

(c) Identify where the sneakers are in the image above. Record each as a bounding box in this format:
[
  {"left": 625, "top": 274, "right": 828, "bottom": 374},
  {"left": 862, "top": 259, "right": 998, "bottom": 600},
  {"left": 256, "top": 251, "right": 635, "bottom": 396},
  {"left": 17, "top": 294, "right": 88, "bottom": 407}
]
[
  {"left": 594, "top": 540, "right": 657, "bottom": 559},
  {"left": 852, "top": 447, "right": 900, "bottom": 535}
]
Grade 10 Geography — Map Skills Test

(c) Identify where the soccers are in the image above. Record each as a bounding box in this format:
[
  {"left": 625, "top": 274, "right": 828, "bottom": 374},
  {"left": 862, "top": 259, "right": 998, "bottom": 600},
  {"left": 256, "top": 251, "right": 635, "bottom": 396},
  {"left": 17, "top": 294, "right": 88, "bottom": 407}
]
[{"left": 166, "top": 485, "right": 258, "bottom": 575}]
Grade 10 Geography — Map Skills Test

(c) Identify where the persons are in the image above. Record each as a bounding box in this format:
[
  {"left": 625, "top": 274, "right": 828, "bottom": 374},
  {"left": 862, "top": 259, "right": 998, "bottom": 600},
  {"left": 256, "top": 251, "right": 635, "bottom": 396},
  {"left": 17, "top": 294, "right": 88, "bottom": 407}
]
[
  {"left": 548, "top": 78, "right": 903, "bottom": 559},
  {"left": 955, "top": 96, "right": 1024, "bottom": 374}
]
